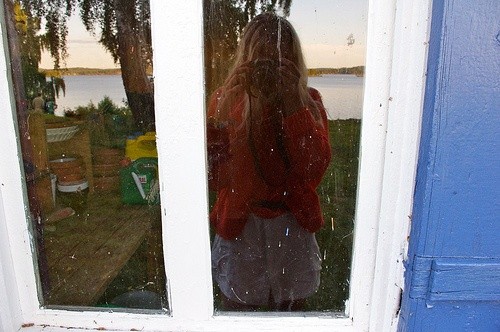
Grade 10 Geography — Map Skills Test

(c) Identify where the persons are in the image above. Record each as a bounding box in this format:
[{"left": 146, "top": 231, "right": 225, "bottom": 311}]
[{"left": 207, "top": 9, "right": 333, "bottom": 312}]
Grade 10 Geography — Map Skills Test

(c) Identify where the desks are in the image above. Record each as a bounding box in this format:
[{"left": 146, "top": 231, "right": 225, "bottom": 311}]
[{"left": 41, "top": 176, "right": 165, "bottom": 308}]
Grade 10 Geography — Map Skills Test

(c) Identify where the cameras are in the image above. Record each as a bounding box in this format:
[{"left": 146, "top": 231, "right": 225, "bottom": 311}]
[{"left": 246, "top": 55, "right": 281, "bottom": 93}]
